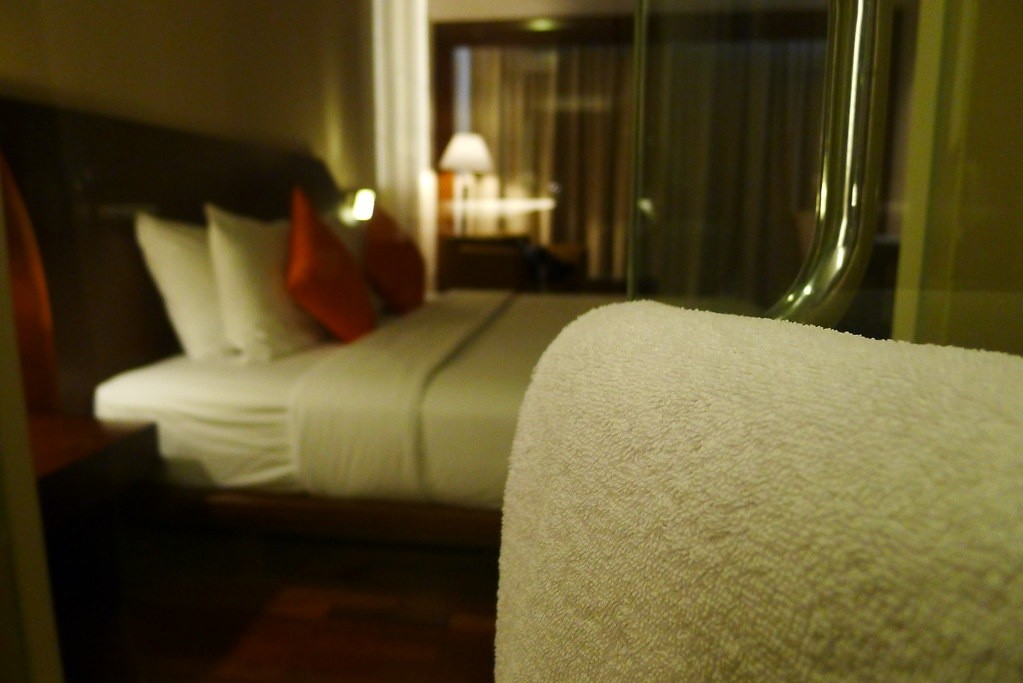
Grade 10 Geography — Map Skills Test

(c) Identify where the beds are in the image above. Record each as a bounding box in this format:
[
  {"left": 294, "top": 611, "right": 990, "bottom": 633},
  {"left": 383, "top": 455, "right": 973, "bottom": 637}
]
[{"left": 0, "top": 91, "right": 766, "bottom": 616}]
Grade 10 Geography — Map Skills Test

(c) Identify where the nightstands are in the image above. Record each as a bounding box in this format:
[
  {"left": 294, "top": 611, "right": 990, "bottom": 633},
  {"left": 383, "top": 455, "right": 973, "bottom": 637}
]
[
  {"left": 436, "top": 231, "right": 539, "bottom": 292},
  {"left": 28, "top": 412, "right": 157, "bottom": 683}
]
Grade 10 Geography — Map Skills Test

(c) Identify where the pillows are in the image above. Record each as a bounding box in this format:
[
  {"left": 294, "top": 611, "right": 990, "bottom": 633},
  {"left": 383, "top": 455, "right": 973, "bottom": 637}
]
[
  {"left": 362, "top": 201, "right": 428, "bottom": 314},
  {"left": 204, "top": 204, "right": 323, "bottom": 364},
  {"left": 285, "top": 190, "right": 378, "bottom": 343},
  {"left": 134, "top": 211, "right": 226, "bottom": 366}
]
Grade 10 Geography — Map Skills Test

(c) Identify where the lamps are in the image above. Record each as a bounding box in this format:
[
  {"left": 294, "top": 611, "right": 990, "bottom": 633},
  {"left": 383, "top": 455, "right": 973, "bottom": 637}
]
[{"left": 439, "top": 131, "right": 492, "bottom": 234}]
